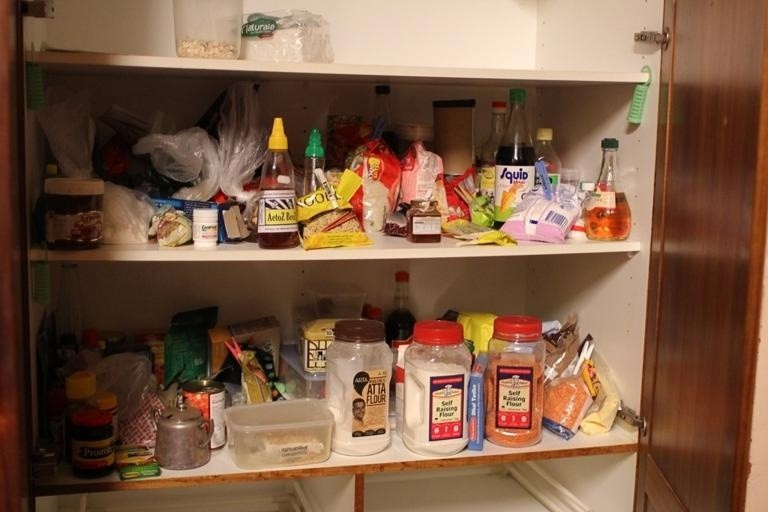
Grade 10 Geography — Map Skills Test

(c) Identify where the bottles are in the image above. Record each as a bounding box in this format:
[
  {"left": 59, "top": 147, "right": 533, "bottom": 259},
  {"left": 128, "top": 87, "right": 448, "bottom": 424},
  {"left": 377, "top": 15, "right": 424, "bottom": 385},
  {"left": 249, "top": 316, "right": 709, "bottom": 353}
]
[
  {"left": 192, "top": 208, "right": 218, "bottom": 250},
  {"left": 584, "top": 137, "right": 631, "bottom": 241},
  {"left": 535, "top": 129, "right": 560, "bottom": 203},
  {"left": 258, "top": 117, "right": 297, "bottom": 248},
  {"left": 483, "top": 314, "right": 545, "bottom": 446},
  {"left": 70, "top": 408, "right": 117, "bottom": 475},
  {"left": 89, "top": 388, "right": 118, "bottom": 445},
  {"left": 476, "top": 102, "right": 505, "bottom": 203},
  {"left": 182, "top": 380, "right": 227, "bottom": 450},
  {"left": 61, "top": 372, "right": 95, "bottom": 417},
  {"left": 495, "top": 87, "right": 535, "bottom": 228},
  {"left": 387, "top": 270, "right": 415, "bottom": 367},
  {"left": 401, "top": 319, "right": 471, "bottom": 454},
  {"left": 302, "top": 126, "right": 326, "bottom": 197},
  {"left": 324, "top": 319, "right": 392, "bottom": 459}
]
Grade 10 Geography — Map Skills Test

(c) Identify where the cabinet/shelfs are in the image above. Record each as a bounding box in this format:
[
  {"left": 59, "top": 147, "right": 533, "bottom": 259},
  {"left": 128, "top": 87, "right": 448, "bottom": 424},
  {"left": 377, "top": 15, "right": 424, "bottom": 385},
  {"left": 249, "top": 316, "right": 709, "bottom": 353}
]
[{"left": 18, "top": 0, "right": 664, "bottom": 512}]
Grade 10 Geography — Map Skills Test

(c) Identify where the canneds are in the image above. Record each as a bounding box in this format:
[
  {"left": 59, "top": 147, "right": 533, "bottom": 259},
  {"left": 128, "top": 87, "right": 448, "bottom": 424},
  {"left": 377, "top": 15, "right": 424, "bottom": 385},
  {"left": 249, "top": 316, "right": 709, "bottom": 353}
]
[
  {"left": 409, "top": 199, "right": 444, "bottom": 242},
  {"left": 44, "top": 177, "right": 107, "bottom": 249}
]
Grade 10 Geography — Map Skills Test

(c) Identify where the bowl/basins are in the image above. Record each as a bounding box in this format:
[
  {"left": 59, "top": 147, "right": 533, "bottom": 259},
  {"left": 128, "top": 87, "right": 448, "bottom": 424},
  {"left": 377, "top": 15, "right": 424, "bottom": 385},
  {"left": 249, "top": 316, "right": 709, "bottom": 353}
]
[{"left": 224, "top": 397, "right": 336, "bottom": 469}]
